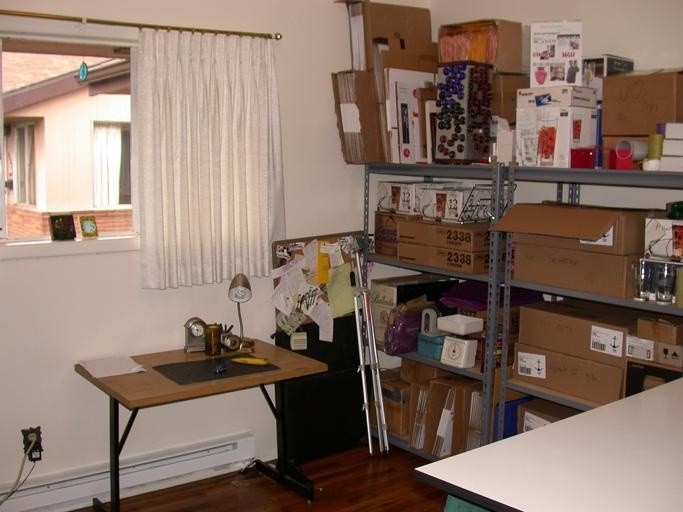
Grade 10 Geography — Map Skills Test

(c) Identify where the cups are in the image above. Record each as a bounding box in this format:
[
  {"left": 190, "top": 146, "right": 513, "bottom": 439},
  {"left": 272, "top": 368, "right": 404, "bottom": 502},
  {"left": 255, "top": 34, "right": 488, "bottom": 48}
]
[
  {"left": 521, "top": 135, "right": 538, "bottom": 165},
  {"left": 650, "top": 238, "right": 675, "bottom": 257},
  {"left": 633, "top": 263, "right": 652, "bottom": 301},
  {"left": 538, "top": 117, "right": 559, "bottom": 163},
  {"left": 205, "top": 324, "right": 221, "bottom": 353},
  {"left": 573, "top": 114, "right": 583, "bottom": 142},
  {"left": 436, "top": 192, "right": 447, "bottom": 218},
  {"left": 390, "top": 184, "right": 401, "bottom": 210},
  {"left": 656, "top": 263, "right": 675, "bottom": 304}
]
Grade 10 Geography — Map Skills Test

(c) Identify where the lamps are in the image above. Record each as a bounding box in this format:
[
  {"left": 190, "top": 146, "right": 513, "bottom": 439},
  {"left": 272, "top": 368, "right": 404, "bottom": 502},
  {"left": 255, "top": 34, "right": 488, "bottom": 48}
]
[{"left": 227, "top": 274, "right": 253, "bottom": 352}]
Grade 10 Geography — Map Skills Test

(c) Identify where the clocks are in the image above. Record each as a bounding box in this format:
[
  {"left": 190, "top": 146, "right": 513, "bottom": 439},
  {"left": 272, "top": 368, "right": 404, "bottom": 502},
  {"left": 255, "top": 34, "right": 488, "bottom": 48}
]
[
  {"left": 79, "top": 216, "right": 98, "bottom": 238},
  {"left": 185, "top": 316, "right": 207, "bottom": 353}
]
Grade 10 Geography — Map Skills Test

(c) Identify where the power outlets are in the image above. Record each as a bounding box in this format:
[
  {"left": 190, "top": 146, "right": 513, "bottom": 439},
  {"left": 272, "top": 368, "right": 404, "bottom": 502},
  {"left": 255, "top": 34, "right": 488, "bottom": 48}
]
[{"left": 23, "top": 426, "right": 41, "bottom": 457}]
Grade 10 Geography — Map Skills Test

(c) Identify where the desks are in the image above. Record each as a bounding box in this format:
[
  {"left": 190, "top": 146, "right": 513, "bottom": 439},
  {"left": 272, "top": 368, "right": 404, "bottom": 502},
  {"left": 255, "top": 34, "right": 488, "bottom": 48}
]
[
  {"left": 71, "top": 337, "right": 329, "bottom": 512},
  {"left": 414, "top": 376, "right": 683, "bottom": 512}
]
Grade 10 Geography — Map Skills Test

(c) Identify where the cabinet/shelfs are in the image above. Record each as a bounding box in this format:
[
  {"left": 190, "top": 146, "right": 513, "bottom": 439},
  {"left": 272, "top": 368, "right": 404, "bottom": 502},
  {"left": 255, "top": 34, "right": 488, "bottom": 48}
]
[
  {"left": 356, "top": 157, "right": 505, "bottom": 468},
  {"left": 498, "top": 160, "right": 683, "bottom": 456}
]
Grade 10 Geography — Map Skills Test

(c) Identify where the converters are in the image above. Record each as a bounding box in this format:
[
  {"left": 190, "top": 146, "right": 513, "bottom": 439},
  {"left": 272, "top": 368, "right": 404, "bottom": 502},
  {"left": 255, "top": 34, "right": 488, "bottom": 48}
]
[{"left": 28, "top": 443, "right": 41, "bottom": 462}]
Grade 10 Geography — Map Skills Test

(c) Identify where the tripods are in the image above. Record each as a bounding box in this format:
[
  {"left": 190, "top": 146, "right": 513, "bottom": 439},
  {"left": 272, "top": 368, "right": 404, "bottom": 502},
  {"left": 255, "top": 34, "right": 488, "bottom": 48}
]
[{"left": 349, "top": 250, "right": 390, "bottom": 459}]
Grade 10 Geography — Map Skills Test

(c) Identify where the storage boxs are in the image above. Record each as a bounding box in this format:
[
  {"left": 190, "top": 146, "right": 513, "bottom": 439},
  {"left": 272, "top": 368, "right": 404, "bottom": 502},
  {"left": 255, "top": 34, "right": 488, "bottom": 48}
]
[
  {"left": 512, "top": 244, "right": 640, "bottom": 301},
  {"left": 393, "top": 220, "right": 491, "bottom": 252},
  {"left": 513, "top": 342, "right": 623, "bottom": 402},
  {"left": 516, "top": 397, "right": 583, "bottom": 443},
  {"left": 394, "top": 243, "right": 489, "bottom": 276},
  {"left": 518, "top": 303, "right": 641, "bottom": 368},
  {"left": 490, "top": 202, "right": 665, "bottom": 254},
  {"left": 365, "top": 368, "right": 409, "bottom": 441},
  {"left": 600, "top": 72, "right": 683, "bottom": 136}
]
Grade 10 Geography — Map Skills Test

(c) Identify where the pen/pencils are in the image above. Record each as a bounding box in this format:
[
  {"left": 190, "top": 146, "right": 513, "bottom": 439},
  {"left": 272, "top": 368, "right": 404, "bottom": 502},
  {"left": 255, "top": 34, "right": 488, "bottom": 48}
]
[{"left": 220, "top": 324, "right": 233, "bottom": 333}]
